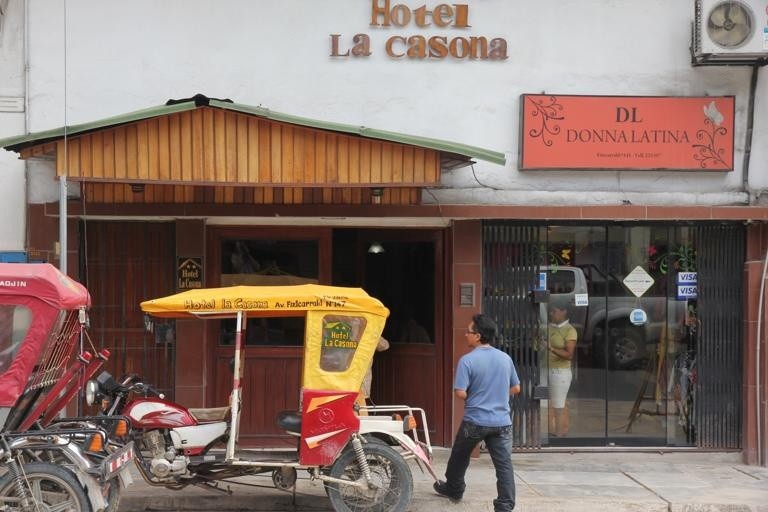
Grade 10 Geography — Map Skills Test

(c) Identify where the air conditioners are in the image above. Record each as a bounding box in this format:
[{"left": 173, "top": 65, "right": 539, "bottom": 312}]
[{"left": 691, "top": 0, "right": 768, "bottom": 61}]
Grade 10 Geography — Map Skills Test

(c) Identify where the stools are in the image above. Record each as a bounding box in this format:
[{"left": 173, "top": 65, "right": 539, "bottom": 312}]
[{"left": 276, "top": 411, "right": 302, "bottom": 437}]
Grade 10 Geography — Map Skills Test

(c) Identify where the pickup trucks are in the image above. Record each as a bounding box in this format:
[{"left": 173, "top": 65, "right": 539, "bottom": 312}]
[{"left": 484, "top": 265, "right": 690, "bottom": 369}]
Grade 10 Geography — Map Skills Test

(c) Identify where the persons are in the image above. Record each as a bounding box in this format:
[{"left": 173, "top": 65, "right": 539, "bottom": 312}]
[
  {"left": 431, "top": 313, "right": 521, "bottom": 511},
  {"left": 347, "top": 317, "right": 390, "bottom": 400},
  {"left": 533, "top": 299, "right": 578, "bottom": 437}
]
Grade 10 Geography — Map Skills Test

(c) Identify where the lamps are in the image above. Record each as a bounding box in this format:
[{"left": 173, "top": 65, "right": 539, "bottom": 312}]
[{"left": 367, "top": 240, "right": 385, "bottom": 254}]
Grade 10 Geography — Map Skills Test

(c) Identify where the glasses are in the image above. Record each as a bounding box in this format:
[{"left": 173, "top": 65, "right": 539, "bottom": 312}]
[{"left": 464, "top": 328, "right": 476, "bottom": 334}]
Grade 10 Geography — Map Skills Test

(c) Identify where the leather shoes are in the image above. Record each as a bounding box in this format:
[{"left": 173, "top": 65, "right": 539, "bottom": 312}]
[{"left": 434, "top": 480, "right": 463, "bottom": 499}]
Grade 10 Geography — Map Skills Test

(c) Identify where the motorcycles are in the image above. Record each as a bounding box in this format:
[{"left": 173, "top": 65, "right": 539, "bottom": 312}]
[
  {"left": 79, "top": 281, "right": 438, "bottom": 511},
  {"left": 0, "top": 259, "right": 136, "bottom": 512}
]
